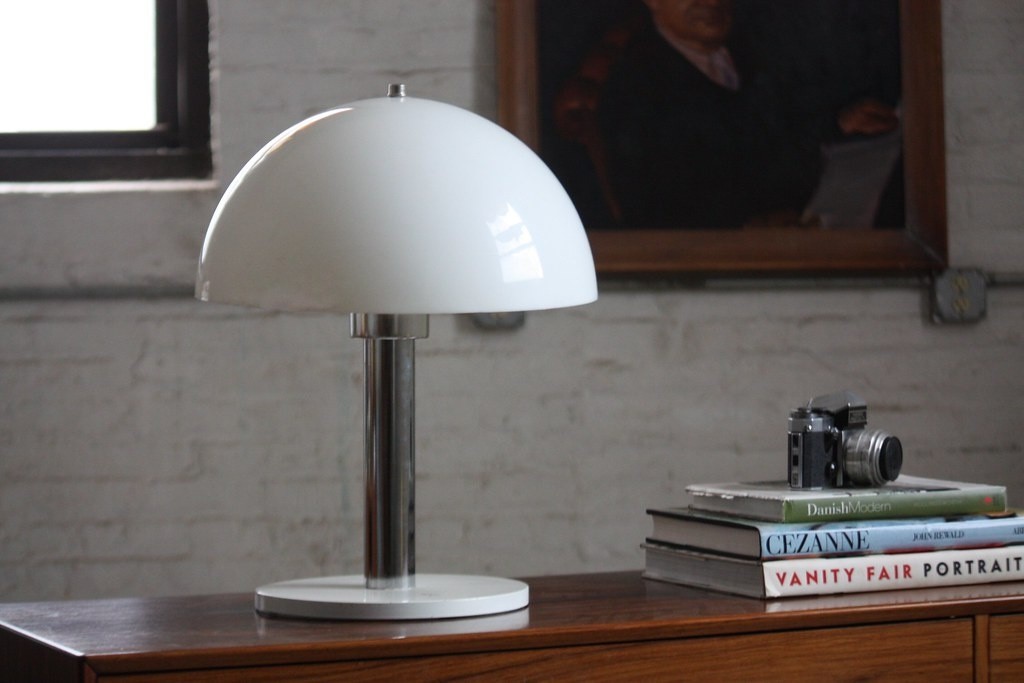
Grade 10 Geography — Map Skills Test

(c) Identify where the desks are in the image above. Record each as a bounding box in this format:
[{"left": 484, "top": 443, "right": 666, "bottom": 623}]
[{"left": 0, "top": 568, "right": 1024, "bottom": 683}]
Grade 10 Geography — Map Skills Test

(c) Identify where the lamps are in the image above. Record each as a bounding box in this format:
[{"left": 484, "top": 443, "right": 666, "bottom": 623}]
[{"left": 193, "top": 83, "right": 601, "bottom": 620}]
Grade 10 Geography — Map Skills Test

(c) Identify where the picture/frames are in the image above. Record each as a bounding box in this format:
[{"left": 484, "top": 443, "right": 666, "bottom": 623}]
[{"left": 495, "top": 0, "right": 949, "bottom": 276}]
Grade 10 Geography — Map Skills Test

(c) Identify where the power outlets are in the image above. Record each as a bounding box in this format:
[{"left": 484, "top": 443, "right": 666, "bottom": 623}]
[
  {"left": 470, "top": 312, "right": 526, "bottom": 330},
  {"left": 933, "top": 265, "right": 987, "bottom": 326}
]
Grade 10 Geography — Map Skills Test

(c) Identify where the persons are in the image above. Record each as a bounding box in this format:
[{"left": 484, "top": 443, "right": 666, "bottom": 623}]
[{"left": 595, "top": 0, "right": 898, "bottom": 228}]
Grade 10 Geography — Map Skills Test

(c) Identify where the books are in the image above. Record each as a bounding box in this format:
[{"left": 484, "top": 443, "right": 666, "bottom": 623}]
[{"left": 640, "top": 473, "right": 1024, "bottom": 598}]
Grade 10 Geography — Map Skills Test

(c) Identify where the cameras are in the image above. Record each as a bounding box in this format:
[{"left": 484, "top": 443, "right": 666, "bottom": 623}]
[{"left": 787, "top": 404, "right": 903, "bottom": 492}]
